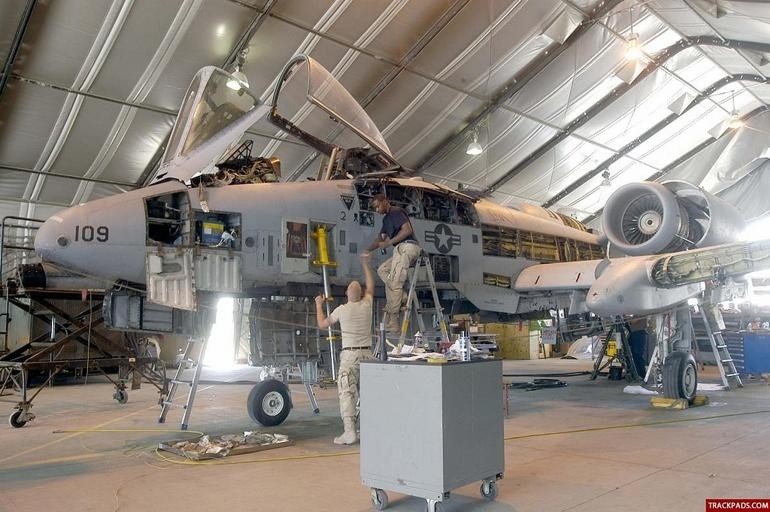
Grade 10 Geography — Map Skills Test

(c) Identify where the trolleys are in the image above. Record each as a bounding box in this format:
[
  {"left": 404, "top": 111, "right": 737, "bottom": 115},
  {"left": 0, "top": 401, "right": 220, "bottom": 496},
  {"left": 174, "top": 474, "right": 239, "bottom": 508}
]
[{"left": 1, "top": 280, "right": 172, "bottom": 430}]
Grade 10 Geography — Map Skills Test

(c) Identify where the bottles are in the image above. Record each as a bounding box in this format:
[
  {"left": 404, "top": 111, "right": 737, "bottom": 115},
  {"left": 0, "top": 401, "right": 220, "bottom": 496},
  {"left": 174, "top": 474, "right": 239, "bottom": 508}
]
[
  {"left": 460, "top": 330, "right": 471, "bottom": 362},
  {"left": 414, "top": 331, "right": 422, "bottom": 353}
]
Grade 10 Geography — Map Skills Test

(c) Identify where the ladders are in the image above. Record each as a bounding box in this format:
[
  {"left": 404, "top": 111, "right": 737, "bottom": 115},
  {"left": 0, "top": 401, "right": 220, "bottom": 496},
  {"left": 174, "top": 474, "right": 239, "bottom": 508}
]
[
  {"left": 353, "top": 248, "right": 450, "bottom": 424},
  {"left": 158, "top": 325, "right": 210, "bottom": 430},
  {"left": 697, "top": 300, "right": 744, "bottom": 391}
]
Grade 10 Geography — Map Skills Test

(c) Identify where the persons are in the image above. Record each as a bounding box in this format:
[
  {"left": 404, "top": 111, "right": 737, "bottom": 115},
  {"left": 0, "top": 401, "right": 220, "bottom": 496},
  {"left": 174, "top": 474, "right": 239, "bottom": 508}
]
[
  {"left": 364, "top": 192, "right": 422, "bottom": 336},
  {"left": 313, "top": 249, "right": 375, "bottom": 445},
  {"left": 625, "top": 316, "right": 649, "bottom": 381}
]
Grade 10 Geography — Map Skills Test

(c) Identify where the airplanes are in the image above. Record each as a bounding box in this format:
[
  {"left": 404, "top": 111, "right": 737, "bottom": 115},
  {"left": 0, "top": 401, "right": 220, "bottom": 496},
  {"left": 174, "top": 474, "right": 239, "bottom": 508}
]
[{"left": 26, "top": 51, "right": 769, "bottom": 427}]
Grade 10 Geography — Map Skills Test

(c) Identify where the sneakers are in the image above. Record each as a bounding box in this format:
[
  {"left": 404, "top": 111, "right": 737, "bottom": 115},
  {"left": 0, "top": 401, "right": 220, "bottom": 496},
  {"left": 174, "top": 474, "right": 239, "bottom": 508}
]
[
  {"left": 380, "top": 319, "right": 403, "bottom": 335},
  {"left": 332, "top": 430, "right": 359, "bottom": 446}
]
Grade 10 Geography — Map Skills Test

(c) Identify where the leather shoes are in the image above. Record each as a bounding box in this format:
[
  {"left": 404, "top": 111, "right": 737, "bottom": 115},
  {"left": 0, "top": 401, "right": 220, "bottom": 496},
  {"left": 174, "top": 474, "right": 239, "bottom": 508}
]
[{"left": 342, "top": 346, "right": 371, "bottom": 351}]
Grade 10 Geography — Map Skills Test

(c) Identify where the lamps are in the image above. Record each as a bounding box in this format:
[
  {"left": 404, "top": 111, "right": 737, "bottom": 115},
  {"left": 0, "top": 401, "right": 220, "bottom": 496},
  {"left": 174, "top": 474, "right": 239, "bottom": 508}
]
[
  {"left": 225, "top": 47, "right": 250, "bottom": 92},
  {"left": 600, "top": 167, "right": 613, "bottom": 187},
  {"left": 466, "top": 125, "right": 483, "bottom": 157}
]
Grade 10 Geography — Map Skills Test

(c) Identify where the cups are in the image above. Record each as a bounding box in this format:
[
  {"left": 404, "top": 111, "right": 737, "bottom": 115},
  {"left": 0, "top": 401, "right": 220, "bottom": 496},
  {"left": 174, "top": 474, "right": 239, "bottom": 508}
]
[{"left": 377, "top": 233, "right": 388, "bottom": 242}]
